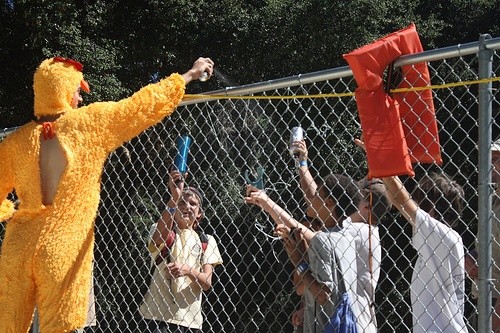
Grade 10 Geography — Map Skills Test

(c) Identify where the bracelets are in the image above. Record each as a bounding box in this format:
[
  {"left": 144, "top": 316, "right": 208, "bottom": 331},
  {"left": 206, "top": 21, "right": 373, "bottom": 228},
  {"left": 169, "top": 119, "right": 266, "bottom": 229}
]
[
  {"left": 295, "top": 262, "right": 311, "bottom": 276},
  {"left": 164, "top": 205, "right": 177, "bottom": 214},
  {"left": 298, "top": 161, "right": 308, "bottom": 166}
]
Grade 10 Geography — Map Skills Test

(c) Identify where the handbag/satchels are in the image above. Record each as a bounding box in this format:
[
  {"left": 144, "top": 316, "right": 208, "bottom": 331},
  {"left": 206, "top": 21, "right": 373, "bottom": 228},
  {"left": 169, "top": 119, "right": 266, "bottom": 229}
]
[{"left": 322, "top": 292, "right": 358, "bottom": 333}]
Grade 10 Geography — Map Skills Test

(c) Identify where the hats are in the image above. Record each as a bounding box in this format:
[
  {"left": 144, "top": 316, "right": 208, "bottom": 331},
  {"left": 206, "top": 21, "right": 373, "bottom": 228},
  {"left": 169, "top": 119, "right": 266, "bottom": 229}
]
[
  {"left": 475, "top": 139, "right": 500, "bottom": 152},
  {"left": 34, "top": 56, "right": 89, "bottom": 117}
]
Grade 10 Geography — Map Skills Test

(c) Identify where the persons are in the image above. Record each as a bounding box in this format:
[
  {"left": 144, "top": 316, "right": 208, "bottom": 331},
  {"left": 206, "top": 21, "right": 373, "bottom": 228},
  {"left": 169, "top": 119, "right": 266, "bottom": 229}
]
[
  {"left": 276, "top": 175, "right": 360, "bottom": 333},
  {"left": 466, "top": 137, "right": 500, "bottom": 333},
  {"left": 245, "top": 139, "right": 393, "bottom": 333},
  {"left": 138, "top": 170, "right": 224, "bottom": 333},
  {"left": 1, "top": 56, "right": 215, "bottom": 333},
  {"left": 356, "top": 139, "right": 469, "bottom": 332}
]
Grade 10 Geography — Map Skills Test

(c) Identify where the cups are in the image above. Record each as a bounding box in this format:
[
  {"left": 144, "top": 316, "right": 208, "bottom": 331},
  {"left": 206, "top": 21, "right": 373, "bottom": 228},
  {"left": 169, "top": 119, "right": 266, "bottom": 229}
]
[
  {"left": 174, "top": 134, "right": 192, "bottom": 173},
  {"left": 289, "top": 127, "right": 304, "bottom": 159},
  {"left": 199, "top": 72, "right": 208, "bottom": 81}
]
